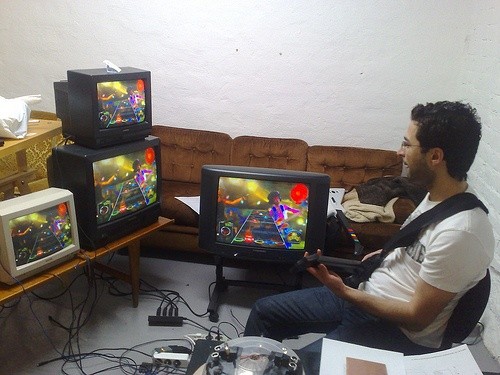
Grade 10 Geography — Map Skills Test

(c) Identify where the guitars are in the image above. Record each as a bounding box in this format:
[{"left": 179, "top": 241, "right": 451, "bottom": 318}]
[
  {"left": 327, "top": 187, "right": 364, "bottom": 255},
  {"left": 289, "top": 253, "right": 491, "bottom": 344}
]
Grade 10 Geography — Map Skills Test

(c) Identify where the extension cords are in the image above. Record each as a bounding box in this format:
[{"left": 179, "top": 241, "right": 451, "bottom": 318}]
[
  {"left": 147, "top": 316, "right": 184, "bottom": 327},
  {"left": 154, "top": 351, "right": 191, "bottom": 369}
]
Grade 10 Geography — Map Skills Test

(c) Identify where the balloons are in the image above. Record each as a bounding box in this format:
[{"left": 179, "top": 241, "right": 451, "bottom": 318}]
[
  {"left": 136, "top": 80, "right": 144, "bottom": 91},
  {"left": 145, "top": 148, "right": 155, "bottom": 165},
  {"left": 291, "top": 184, "right": 308, "bottom": 204},
  {"left": 57, "top": 204, "right": 66, "bottom": 216}
]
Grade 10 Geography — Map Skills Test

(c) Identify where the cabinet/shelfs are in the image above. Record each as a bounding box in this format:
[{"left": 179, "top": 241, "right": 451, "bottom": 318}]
[{"left": 0, "top": 120, "right": 174, "bottom": 308}]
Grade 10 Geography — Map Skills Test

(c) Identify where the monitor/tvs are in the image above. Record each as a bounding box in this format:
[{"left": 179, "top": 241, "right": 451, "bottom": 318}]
[
  {"left": 0, "top": 186, "right": 80, "bottom": 287},
  {"left": 52, "top": 66, "right": 153, "bottom": 148},
  {"left": 197, "top": 165, "right": 331, "bottom": 262},
  {"left": 46, "top": 135, "right": 159, "bottom": 250}
]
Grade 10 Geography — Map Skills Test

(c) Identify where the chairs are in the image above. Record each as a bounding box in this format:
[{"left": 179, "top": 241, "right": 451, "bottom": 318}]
[{"left": 440, "top": 268, "right": 490, "bottom": 350}]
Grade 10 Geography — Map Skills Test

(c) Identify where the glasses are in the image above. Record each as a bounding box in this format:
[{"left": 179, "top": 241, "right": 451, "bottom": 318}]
[{"left": 401, "top": 141, "right": 421, "bottom": 149}]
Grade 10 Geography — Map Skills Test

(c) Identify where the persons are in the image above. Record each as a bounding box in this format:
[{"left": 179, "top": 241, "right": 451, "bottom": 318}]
[
  {"left": 127, "top": 87, "right": 142, "bottom": 121},
  {"left": 132, "top": 159, "right": 152, "bottom": 203},
  {"left": 245, "top": 102, "right": 494, "bottom": 375},
  {"left": 46, "top": 215, "right": 65, "bottom": 247},
  {"left": 267, "top": 191, "right": 300, "bottom": 249}
]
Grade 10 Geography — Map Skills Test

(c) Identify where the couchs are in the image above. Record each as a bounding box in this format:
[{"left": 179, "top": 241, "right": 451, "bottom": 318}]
[{"left": 142, "top": 126, "right": 417, "bottom": 276}]
